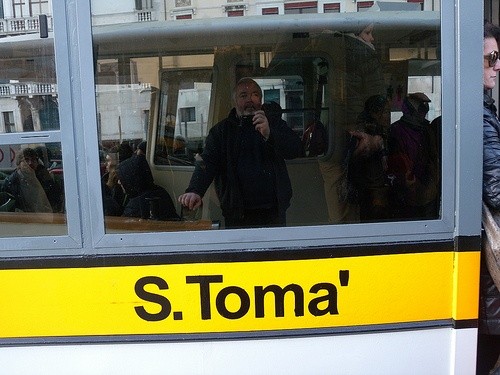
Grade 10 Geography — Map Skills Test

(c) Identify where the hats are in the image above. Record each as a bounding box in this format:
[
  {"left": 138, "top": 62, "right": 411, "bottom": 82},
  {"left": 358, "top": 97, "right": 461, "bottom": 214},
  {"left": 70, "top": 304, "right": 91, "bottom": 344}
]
[{"left": 137, "top": 141, "right": 147, "bottom": 153}]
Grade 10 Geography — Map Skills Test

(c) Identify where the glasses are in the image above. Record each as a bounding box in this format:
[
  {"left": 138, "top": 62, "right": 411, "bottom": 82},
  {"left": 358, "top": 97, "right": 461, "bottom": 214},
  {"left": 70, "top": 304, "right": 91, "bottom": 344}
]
[
  {"left": 484, "top": 50, "right": 500, "bottom": 67},
  {"left": 19, "top": 158, "right": 38, "bottom": 164}
]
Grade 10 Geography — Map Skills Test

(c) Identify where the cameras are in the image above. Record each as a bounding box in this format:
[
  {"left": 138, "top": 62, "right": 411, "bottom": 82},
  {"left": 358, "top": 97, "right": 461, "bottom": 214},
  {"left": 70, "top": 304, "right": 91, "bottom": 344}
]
[{"left": 240, "top": 114, "right": 256, "bottom": 130}]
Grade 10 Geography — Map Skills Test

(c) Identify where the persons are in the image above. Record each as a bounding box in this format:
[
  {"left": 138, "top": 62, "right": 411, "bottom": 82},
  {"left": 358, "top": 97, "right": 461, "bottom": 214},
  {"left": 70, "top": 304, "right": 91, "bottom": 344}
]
[
  {"left": 2, "top": 137, "right": 185, "bottom": 219},
  {"left": 262, "top": 20, "right": 500, "bottom": 375},
  {"left": 178, "top": 77, "right": 302, "bottom": 228}
]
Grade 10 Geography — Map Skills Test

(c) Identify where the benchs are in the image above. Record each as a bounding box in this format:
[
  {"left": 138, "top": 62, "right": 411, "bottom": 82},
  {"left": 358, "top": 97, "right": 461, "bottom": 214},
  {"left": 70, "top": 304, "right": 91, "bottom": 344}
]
[{"left": 0, "top": 212, "right": 221, "bottom": 238}]
